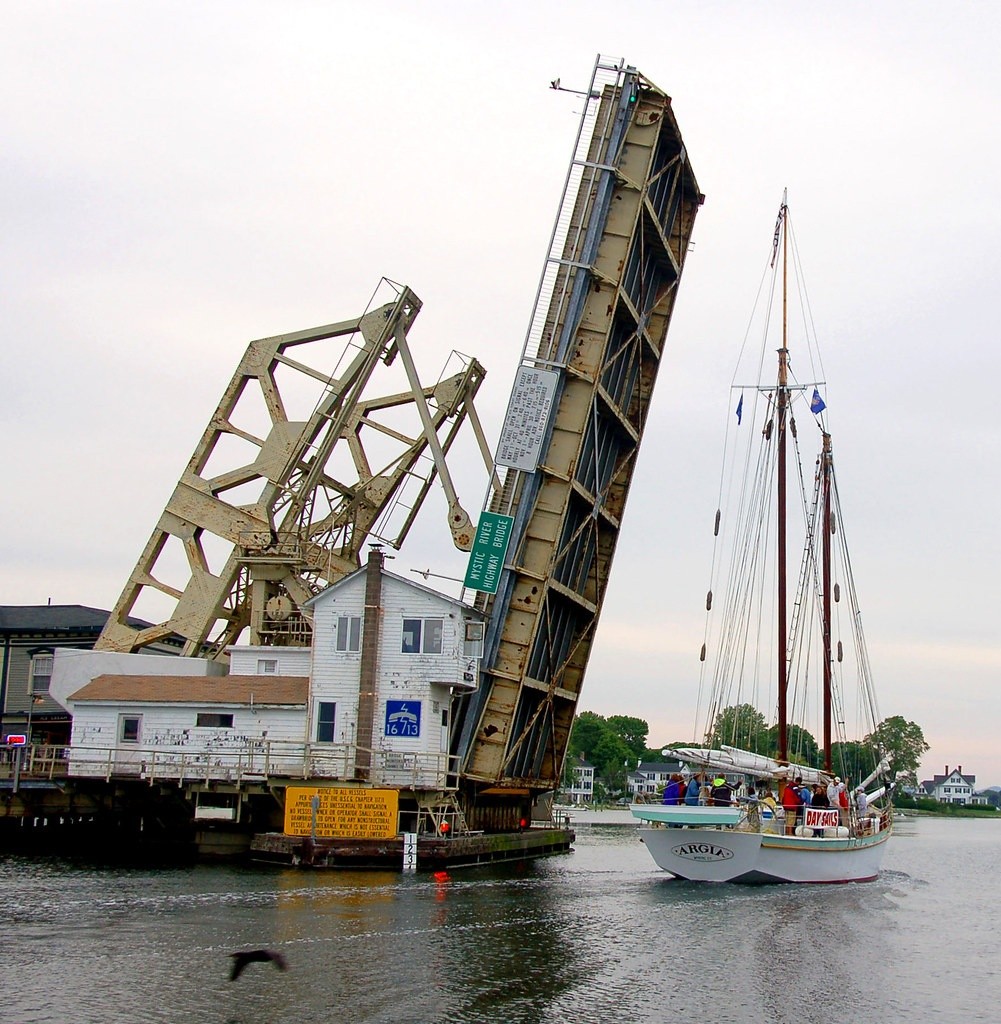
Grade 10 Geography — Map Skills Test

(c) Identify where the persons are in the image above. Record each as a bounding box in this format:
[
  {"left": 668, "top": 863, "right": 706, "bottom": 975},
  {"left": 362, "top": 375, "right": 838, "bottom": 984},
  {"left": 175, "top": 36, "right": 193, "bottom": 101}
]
[
  {"left": 62, "top": 748, "right": 70, "bottom": 772},
  {"left": 37, "top": 739, "right": 52, "bottom": 771},
  {"left": 660, "top": 774, "right": 867, "bottom": 838}
]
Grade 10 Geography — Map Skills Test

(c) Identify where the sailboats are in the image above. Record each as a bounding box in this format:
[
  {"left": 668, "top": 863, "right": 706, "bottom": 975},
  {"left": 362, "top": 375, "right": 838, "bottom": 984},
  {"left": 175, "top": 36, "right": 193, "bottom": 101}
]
[{"left": 627, "top": 182, "right": 905, "bottom": 886}]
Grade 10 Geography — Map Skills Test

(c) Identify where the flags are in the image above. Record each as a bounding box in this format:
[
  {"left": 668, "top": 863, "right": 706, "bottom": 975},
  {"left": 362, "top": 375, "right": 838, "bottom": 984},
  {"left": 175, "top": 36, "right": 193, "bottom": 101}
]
[
  {"left": 810, "top": 389, "right": 825, "bottom": 416},
  {"left": 735, "top": 394, "right": 742, "bottom": 425}
]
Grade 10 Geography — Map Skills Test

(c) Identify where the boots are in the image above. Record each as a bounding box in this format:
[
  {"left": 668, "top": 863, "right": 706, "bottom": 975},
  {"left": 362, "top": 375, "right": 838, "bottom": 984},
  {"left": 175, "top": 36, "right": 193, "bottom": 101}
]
[{"left": 785, "top": 827, "right": 793, "bottom": 836}]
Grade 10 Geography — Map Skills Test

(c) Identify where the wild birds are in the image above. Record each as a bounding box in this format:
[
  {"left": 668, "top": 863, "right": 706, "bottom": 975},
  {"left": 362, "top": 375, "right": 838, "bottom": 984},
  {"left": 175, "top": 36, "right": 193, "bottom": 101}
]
[{"left": 226, "top": 949, "right": 288, "bottom": 983}]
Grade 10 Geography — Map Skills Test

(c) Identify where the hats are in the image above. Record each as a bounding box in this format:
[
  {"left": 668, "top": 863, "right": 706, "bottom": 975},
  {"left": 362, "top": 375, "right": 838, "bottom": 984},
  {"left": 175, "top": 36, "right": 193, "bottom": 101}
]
[
  {"left": 835, "top": 777, "right": 840, "bottom": 783},
  {"left": 857, "top": 787, "right": 864, "bottom": 792}
]
